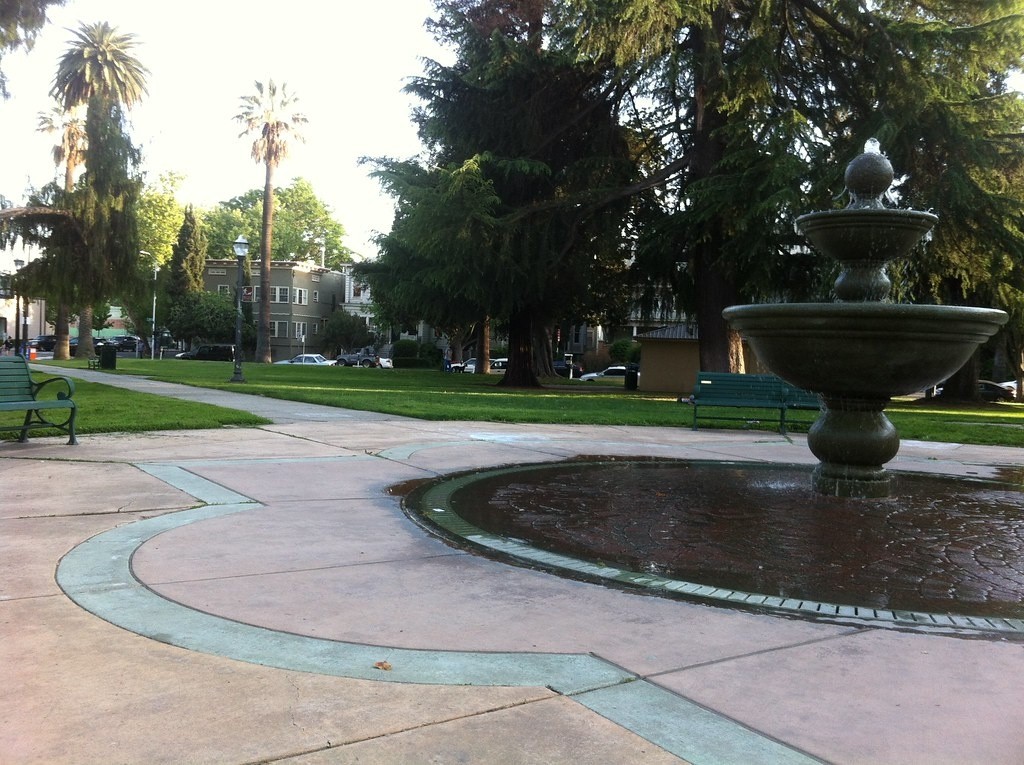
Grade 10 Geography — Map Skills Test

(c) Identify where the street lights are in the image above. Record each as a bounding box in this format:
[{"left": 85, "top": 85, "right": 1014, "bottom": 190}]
[
  {"left": 230, "top": 233, "right": 250, "bottom": 386},
  {"left": 139, "top": 250, "right": 157, "bottom": 360},
  {"left": 12, "top": 258, "right": 25, "bottom": 357}
]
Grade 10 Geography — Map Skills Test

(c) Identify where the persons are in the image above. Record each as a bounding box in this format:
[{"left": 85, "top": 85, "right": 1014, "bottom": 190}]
[
  {"left": 138, "top": 340, "right": 145, "bottom": 359},
  {"left": 2, "top": 336, "right": 12, "bottom": 355},
  {"left": 444, "top": 347, "right": 453, "bottom": 374}
]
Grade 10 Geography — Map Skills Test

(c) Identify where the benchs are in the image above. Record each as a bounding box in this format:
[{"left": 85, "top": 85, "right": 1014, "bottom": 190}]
[
  {"left": 86, "top": 355, "right": 100, "bottom": 370},
  {"left": 0, "top": 354, "right": 79, "bottom": 445},
  {"left": 688, "top": 373, "right": 821, "bottom": 436}
]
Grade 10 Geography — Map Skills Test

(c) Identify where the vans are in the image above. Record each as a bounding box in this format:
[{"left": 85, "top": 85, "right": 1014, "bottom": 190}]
[
  {"left": 490, "top": 358, "right": 509, "bottom": 374},
  {"left": 175, "top": 343, "right": 236, "bottom": 363}
]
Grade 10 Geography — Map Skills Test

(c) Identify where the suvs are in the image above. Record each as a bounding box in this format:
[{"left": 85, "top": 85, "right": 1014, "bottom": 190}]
[
  {"left": 337, "top": 347, "right": 380, "bottom": 369},
  {"left": 447, "top": 357, "right": 477, "bottom": 373}
]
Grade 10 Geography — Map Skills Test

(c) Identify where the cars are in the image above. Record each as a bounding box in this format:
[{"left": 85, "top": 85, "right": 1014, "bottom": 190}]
[
  {"left": 26, "top": 334, "right": 140, "bottom": 358},
  {"left": 272, "top": 353, "right": 337, "bottom": 367},
  {"left": 376, "top": 357, "right": 394, "bottom": 368},
  {"left": 553, "top": 360, "right": 584, "bottom": 379},
  {"left": 935, "top": 379, "right": 1021, "bottom": 403},
  {"left": 578, "top": 366, "right": 640, "bottom": 388}
]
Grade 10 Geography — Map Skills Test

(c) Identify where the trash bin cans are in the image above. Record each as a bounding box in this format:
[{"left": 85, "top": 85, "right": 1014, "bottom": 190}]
[{"left": 99, "top": 342, "right": 116, "bottom": 370}]
